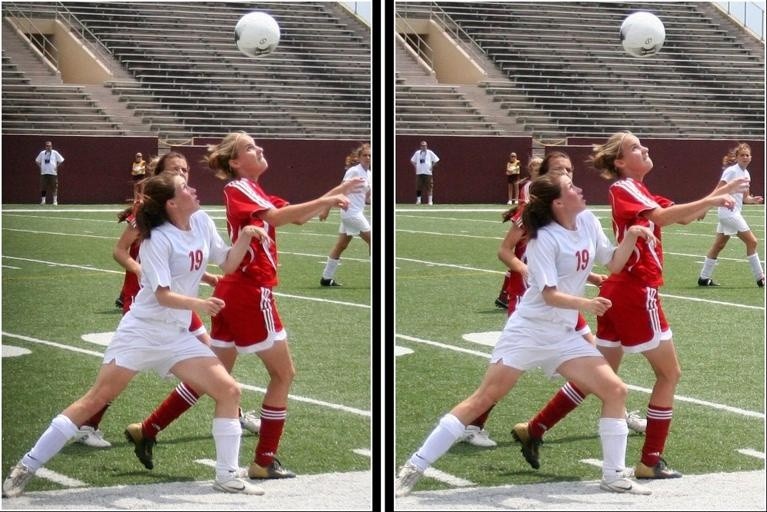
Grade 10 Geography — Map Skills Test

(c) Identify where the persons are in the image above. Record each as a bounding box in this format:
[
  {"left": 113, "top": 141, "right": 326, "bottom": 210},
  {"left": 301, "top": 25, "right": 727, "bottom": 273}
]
[
  {"left": 319, "top": 146, "right": 371, "bottom": 287},
  {"left": 3, "top": 170, "right": 275, "bottom": 500},
  {"left": 123, "top": 131, "right": 366, "bottom": 482},
  {"left": 494, "top": 158, "right": 544, "bottom": 308},
  {"left": 506, "top": 153, "right": 520, "bottom": 205},
  {"left": 75, "top": 152, "right": 262, "bottom": 448},
  {"left": 116, "top": 158, "right": 161, "bottom": 309},
  {"left": 35, "top": 142, "right": 64, "bottom": 207},
  {"left": 512, "top": 130, "right": 751, "bottom": 478},
  {"left": 699, "top": 143, "right": 766, "bottom": 288},
  {"left": 410, "top": 142, "right": 440, "bottom": 206},
  {"left": 462, "top": 151, "right": 647, "bottom": 447},
  {"left": 132, "top": 153, "right": 146, "bottom": 204},
  {"left": 394, "top": 169, "right": 661, "bottom": 497}
]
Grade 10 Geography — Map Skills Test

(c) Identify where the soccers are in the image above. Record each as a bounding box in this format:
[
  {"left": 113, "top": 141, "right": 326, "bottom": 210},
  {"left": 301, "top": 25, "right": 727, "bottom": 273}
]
[
  {"left": 620, "top": 12, "right": 667, "bottom": 58},
  {"left": 233, "top": 11, "right": 280, "bottom": 58}
]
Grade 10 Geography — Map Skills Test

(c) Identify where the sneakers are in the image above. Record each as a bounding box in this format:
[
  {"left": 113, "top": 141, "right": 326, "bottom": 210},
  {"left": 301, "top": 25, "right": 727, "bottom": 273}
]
[
  {"left": 599, "top": 476, "right": 651, "bottom": 495},
  {"left": 697, "top": 277, "right": 721, "bottom": 287},
  {"left": 461, "top": 426, "right": 496, "bottom": 447},
  {"left": 756, "top": 277, "right": 765, "bottom": 290},
  {"left": 74, "top": 425, "right": 114, "bottom": 451},
  {"left": 633, "top": 463, "right": 682, "bottom": 479},
  {"left": 512, "top": 423, "right": 542, "bottom": 470},
  {"left": 115, "top": 295, "right": 125, "bottom": 310},
  {"left": 2, "top": 457, "right": 34, "bottom": 498},
  {"left": 124, "top": 423, "right": 159, "bottom": 471},
  {"left": 626, "top": 414, "right": 649, "bottom": 432},
  {"left": 242, "top": 410, "right": 265, "bottom": 434},
  {"left": 247, "top": 460, "right": 298, "bottom": 480},
  {"left": 393, "top": 461, "right": 423, "bottom": 498},
  {"left": 493, "top": 296, "right": 511, "bottom": 312},
  {"left": 319, "top": 277, "right": 343, "bottom": 288},
  {"left": 213, "top": 473, "right": 266, "bottom": 497}
]
